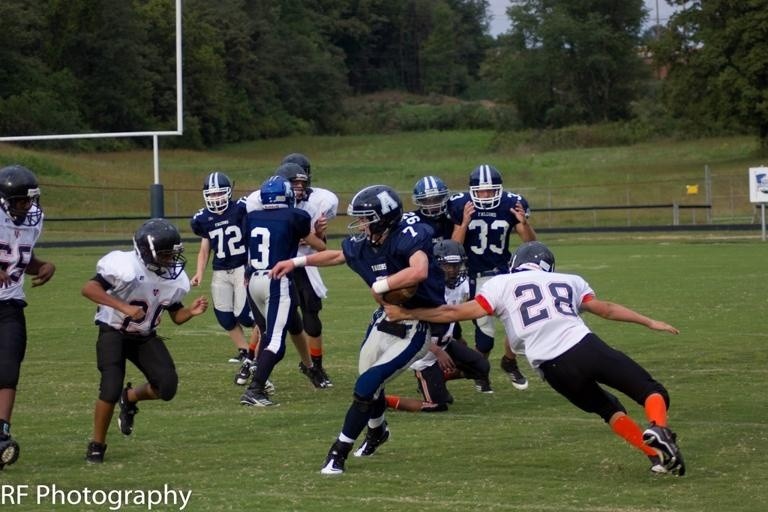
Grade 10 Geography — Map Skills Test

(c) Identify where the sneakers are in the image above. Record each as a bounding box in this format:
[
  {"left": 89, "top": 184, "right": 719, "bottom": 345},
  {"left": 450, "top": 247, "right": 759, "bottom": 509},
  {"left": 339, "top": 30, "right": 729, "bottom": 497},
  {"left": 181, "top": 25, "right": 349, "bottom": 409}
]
[
  {"left": 641, "top": 421, "right": 684, "bottom": 478},
  {"left": 86, "top": 441, "right": 107, "bottom": 466},
  {"left": 228, "top": 348, "right": 280, "bottom": 407},
  {"left": 320, "top": 442, "right": 349, "bottom": 475},
  {"left": 354, "top": 421, "right": 390, "bottom": 458},
  {"left": 298, "top": 361, "right": 333, "bottom": 388},
  {"left": 500, "top": 357, "right": 528, "bottom": 391},
  {"left": 118, "top": 383, "right": 137, "bottom": 435},
  {"left": 0, "top": 432, "right": 19, "bottom": 471},
  {"left": 474, "top": 378, "right": 493, "bottom": 394}
]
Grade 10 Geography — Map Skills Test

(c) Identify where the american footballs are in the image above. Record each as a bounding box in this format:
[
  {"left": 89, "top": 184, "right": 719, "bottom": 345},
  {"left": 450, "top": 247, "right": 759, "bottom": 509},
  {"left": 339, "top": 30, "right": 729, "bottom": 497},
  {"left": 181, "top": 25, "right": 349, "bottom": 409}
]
[{"left": 384, "top": 285, "right": 419, "bottom": 304}]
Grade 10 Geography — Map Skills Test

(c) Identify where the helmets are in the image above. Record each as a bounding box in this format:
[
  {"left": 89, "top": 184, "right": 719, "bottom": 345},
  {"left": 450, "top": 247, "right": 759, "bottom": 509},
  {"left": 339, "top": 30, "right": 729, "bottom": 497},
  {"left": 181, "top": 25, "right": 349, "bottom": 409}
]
[
  {"left": 509, "top": 241, "right": 554, "bottom": 273},
  {"left": 432, "top": 240, "right": 468, "bottom": 289},
  {"left": 203, "top": 172, "right": 233, "bottom": 215},
  {"left": 347, "top": 185, "right": 403, "bottom": 244},
  {"left": 0, "top": 165, "right": 44, "bottom": 227},
  {"left": 261, "top": 154, "right": 311, "bottom": 210},
  {"left": 134, "top": 218, "right": 187, "bottom": 279},
  {"left": 469, "top": 164, "right": 504, "bottom": 210},
  {"left": 411, "top": 176, "right": 450, "bottom": 217}
]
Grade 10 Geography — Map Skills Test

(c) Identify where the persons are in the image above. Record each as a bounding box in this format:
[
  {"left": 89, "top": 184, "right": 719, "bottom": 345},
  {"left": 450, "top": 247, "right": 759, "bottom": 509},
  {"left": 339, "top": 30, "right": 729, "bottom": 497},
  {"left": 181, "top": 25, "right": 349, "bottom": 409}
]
[
  {"left": 187, "top": 152, "right": 689, "bottom": 478},
  {"left": 78, "top": 215, "right": 210, "bottom": 465},
  {"left": 0, "top": 163, "right": 58, "bottom": 474}
]
